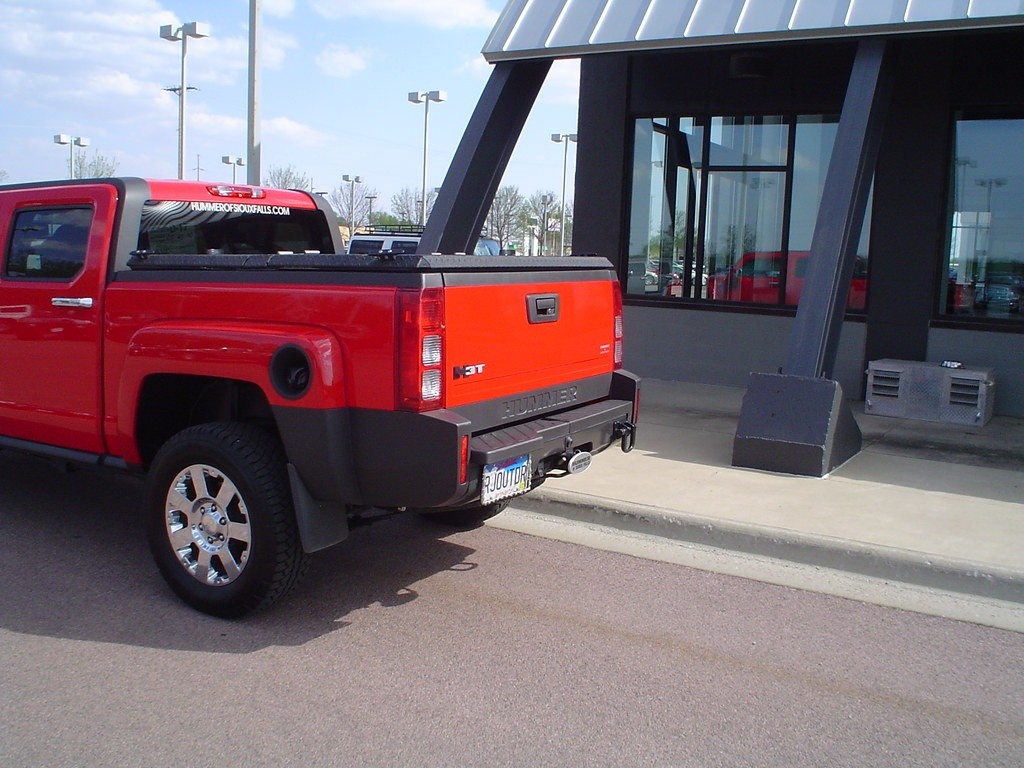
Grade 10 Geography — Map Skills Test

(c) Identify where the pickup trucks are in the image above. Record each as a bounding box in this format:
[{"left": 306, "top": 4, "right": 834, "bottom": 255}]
[
  {"left": 1, "top": 171, "right": 647, "bottom": 623},
  {"left": 705, "top": 251, "right": 968, "bottom": 313}
]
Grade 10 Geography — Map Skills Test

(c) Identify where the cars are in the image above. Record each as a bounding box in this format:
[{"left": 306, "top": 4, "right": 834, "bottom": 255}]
[
  {"left": 645, "top": 258, "right": 708, "bottom": 288},
  {"left": 948, "top": 269, "right": 1024, "bottom": 316}
]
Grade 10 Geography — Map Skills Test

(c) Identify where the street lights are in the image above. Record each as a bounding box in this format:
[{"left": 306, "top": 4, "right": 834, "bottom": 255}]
[
  {"left": 408, "top": 90, "right": 447, "bottom": 230},
  {"left": 158, "top": 21, "right": 211, "bottom": 180},
  {"left": 551, "top": 133, "right": 579, "bottom": 256},
  {"left": 342, "top": 174, "right": 363, "bottom": 236},
  {"left": 221, "top": 156, "right": 245, "bottom": 185},
  {"left": 365, "top": 197, "right": 378, "bottom": 234},
  {"left": 53, "top": 133, "right": 89, "bottom": 179}
]
